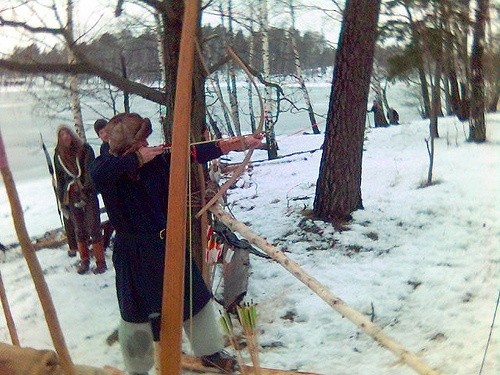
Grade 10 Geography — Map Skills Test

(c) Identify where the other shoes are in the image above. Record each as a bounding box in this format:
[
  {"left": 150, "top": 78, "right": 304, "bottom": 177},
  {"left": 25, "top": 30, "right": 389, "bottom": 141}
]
[
  {"left": 77, "top": 259, "right": 90, "bottom": 273},
  {"left": 202, "top": 350, "right": 237, "bottom": 371},
  {"left": 94, "top": 258, "right": 108, "bottom": 275},
  {"left": 68, "top": 249, "right": 78, "bottom": 257}
]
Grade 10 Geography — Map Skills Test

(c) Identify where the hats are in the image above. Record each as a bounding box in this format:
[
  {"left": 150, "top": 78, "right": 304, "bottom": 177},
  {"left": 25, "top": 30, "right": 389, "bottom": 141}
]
[{"left": 105, "top": 112, "right": 153, "bottom": 155}]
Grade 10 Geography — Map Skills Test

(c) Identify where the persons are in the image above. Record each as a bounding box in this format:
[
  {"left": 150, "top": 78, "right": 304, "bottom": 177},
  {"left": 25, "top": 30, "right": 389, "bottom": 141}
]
[
  {"left": 88, "top": 113, "right": 265, "bottom": 375},
  {"left": 94, "top": 118, "right": 110, "bottom": 154},
  {"left": 53, "top": 125, "right": 109, "bottom": 275}
]
